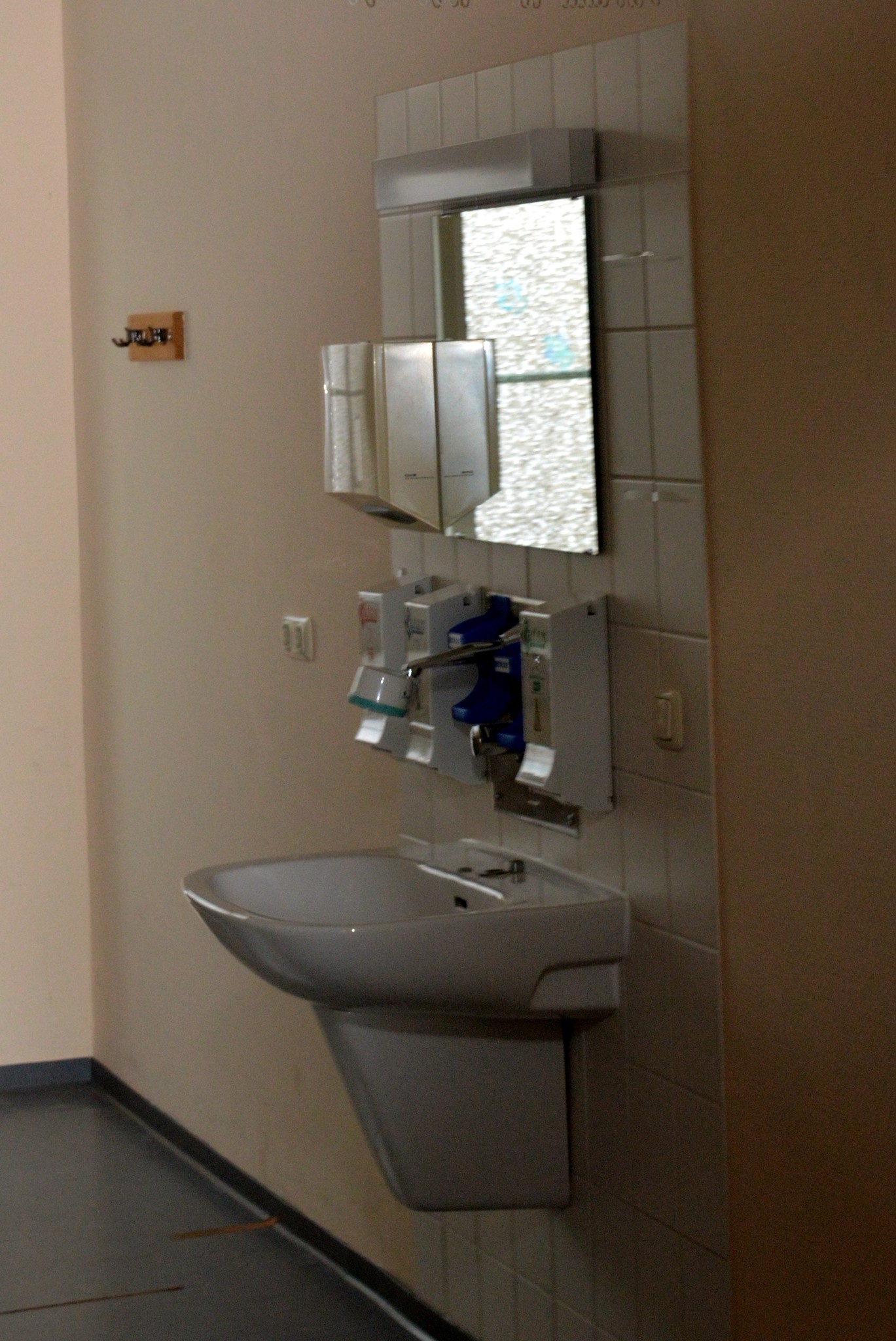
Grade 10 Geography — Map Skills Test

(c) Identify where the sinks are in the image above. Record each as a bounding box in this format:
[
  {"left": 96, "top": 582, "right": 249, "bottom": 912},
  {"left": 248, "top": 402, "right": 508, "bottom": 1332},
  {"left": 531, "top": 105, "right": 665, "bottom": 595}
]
[{"left": 177, "top": 840, "right": 633, "bottom": 1021}]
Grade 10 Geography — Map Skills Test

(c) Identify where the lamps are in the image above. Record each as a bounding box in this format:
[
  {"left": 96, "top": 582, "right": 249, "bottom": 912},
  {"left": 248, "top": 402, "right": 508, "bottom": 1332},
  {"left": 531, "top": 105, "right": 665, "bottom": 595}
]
[{"left": 373, "top": 124, "right": 601, "bottom": 215}]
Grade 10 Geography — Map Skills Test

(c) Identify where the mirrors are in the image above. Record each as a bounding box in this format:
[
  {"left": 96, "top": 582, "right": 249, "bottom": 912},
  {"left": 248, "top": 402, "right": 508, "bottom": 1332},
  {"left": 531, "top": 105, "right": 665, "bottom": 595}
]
[{"left": 430, "top": 193, "right": 613, "bottom": 561}]
